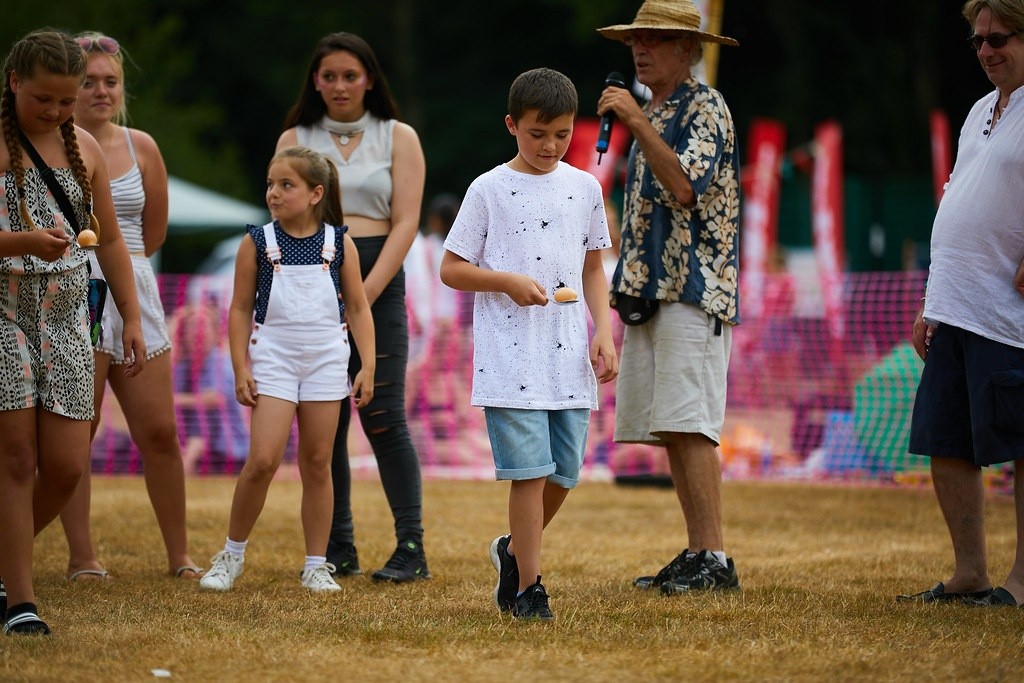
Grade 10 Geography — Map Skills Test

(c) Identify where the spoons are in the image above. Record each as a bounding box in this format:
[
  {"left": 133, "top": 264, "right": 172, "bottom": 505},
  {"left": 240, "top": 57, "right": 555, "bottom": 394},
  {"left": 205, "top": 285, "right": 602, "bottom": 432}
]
[
  {"left": 545, "top": 297, "right": 580, "bottom": 306},
  {"left": 63, "top": 238, "right": 100, "bottom": 251}
]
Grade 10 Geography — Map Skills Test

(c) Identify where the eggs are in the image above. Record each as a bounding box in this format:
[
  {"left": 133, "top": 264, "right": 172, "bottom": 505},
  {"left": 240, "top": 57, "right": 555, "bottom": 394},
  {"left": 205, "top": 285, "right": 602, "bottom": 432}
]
[
  {"left": 555, "top": 287, "right": 577, "bottom": 302},
  {"left": 78, "top": 229, "right": 97, "bottom": 247}
]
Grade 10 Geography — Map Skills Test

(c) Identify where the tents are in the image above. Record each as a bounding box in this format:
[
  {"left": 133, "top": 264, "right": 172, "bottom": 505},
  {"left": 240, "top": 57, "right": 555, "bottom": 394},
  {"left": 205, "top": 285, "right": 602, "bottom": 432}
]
[{"left": 149, "top": 176, "right": 270, "bottom": 270}]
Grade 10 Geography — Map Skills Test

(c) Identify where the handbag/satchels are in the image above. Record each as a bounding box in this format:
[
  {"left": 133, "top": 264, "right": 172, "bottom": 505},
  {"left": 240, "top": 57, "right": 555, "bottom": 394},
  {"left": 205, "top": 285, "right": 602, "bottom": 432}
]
[{"left": 87, "top": 278, "right": 108, "bottom": 347}]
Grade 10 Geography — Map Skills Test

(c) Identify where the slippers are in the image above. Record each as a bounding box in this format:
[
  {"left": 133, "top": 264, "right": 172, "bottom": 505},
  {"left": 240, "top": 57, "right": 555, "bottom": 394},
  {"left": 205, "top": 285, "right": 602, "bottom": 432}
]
[
  {"left": 176, "top": 566, "right": 204, "bottom": 579},
  {"left": 69, "top": 570, "right": 108, "bottom": 580}
]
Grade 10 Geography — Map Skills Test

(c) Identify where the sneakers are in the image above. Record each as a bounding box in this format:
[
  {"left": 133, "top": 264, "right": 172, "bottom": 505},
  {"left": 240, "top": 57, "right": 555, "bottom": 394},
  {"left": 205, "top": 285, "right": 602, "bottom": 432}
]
[
  {"left": 199, "top": 551, "right": 244, "bottom": 592},
  {"left": 632, "top": 548, "right": 692, "bottom": 590},
  {"left": 514, "top": 575, "right": 554, "bottom": 621},
  {"left": 3, "top": 603, "right": 51, "bottom": 635},
  {"left": 301, "top": 563, "right": 342, "bottom": 592},
  {"left": 0, "top": 577, "right": 8, "bottom": 625},
  {"left": 489, "top": 534, "right": 519, "bottom": 614},
  {"left": 659, "top": 550, "right": 741, "bottom": 596},
  {"left": 371, "top": 540, "right": 431, "bottom": 584},
  {"left": 300, "top": 542, "right": 363, "bottom": 579}
]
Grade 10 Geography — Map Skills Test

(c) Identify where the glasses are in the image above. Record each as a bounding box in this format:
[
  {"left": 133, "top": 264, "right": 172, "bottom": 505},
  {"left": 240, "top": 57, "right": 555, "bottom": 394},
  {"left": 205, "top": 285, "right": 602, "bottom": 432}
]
[
  {"left": 619, "top": 32, "right": 685, "bottom": 46},
  {"left": 73, "top": 36, "right": 120, "bottom": 59},
  {"left": 966, "top": 29, "right": 1023, "bottom": 50}
]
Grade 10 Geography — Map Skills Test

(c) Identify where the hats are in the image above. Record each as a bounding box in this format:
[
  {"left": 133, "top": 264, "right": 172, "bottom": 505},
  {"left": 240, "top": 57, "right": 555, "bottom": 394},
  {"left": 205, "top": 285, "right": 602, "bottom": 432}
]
[{"left": 595, "top": 0, "right": 740, "bottom": 47}]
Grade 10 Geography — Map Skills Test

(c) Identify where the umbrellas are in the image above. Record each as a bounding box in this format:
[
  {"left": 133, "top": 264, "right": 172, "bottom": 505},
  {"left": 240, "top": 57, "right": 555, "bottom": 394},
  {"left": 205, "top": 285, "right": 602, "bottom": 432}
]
[{"left": 854, "top": 344, "right": 1007, "bottom": 473}]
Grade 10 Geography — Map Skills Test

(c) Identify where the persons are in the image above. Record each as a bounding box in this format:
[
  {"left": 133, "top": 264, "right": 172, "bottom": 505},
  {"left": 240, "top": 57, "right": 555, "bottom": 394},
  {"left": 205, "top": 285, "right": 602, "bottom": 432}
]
[
  {"left": 439, "top": 68, "right": 621, "bottom": 622},
  {"left": 0, "top": 30, "right": 146, "bottom": 637},
  {"left": 593, "top": 0, "right": 739, "bottom": 595},
  {"left": 275, "top": 32, "right": 424, "bottom": 585},
  {"left": 896, "top": 0, "right": 1023, "bottom": 607},
  {"left": 58, "top": 34, "right": 209, "bottom": 579},
  {"left": 199, "top": 147, "right": 376, "bottom": 591},
  {"left": 170, "top": 305, "right": 249, "bottom": 478},
  {"left": 401, "top": 195, "right": 493, "bottom": 465}
]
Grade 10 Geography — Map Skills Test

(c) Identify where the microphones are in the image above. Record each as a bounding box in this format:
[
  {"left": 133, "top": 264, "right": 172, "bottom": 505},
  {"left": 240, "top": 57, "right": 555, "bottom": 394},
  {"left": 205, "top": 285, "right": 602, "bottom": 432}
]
[{"left": 595, "top": 72, "right": 626, "bottom": 165}]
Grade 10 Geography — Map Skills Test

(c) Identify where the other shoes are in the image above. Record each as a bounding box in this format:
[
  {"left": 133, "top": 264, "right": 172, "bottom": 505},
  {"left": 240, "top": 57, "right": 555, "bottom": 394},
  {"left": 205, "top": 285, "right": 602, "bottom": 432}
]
[
  {"left": 976, "top": 587, "right": 1024, "bottom": 610},
  {"left": 895, "top": 582, "right": 993, "bottom": 602}
]
